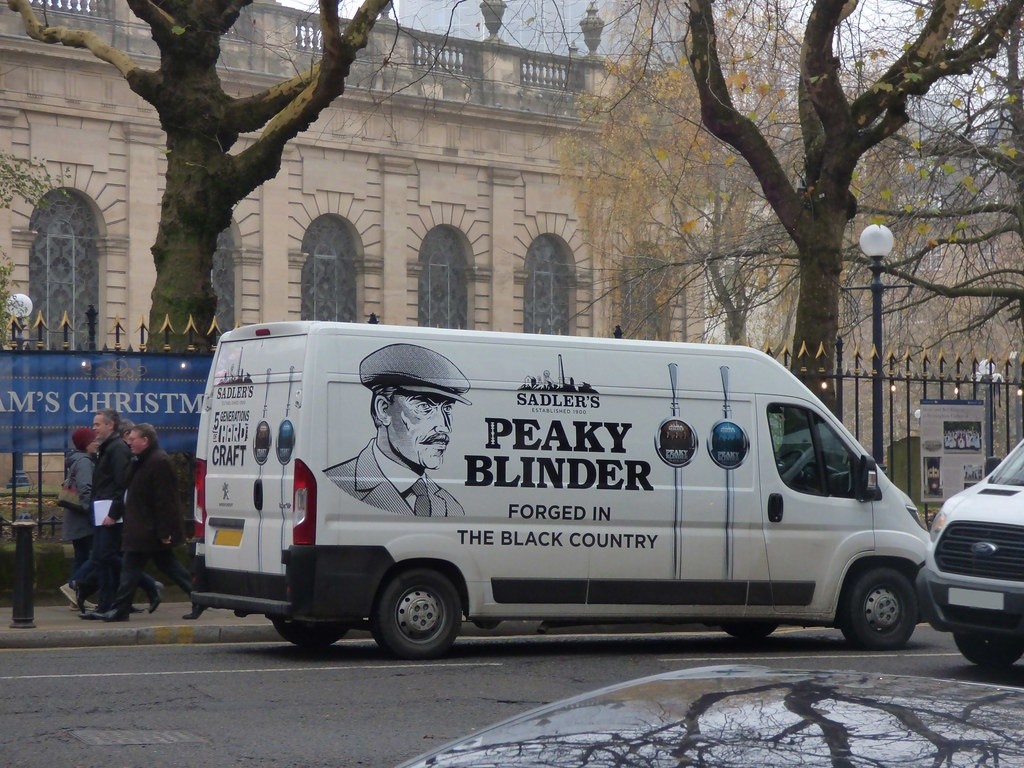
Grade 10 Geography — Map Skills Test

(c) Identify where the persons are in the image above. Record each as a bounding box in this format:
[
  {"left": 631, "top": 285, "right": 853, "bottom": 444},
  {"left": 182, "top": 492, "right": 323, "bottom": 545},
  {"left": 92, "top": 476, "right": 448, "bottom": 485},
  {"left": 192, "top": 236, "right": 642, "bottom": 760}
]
[{"left": 58, "top": 407, "right": 210, "bottom": 622}]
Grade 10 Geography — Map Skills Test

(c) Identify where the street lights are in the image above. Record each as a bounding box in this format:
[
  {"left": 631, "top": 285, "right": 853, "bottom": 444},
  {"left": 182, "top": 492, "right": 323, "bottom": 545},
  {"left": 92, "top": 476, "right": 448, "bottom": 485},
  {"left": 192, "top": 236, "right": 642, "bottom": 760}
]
[
  {"left": 979, "top": 359, "right": 996, "bottom": 460},
  {"left": 860, "top": 223, "right": 895, "bottom": 476},
  {"left": 7, "top": 292, "right": 34, "bottom": 490}
]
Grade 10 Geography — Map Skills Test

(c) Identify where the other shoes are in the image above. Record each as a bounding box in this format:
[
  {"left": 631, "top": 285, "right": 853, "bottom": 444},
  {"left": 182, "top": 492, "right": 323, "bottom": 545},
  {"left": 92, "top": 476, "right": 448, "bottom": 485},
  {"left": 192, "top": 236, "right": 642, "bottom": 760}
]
[
  {"left": 129, "top": 606, "right": 144, "bottom": 613},
  {"left": 80, "top": 608, "right": 104, "bottom": 619},
  {"left": 148, "top": 581, "right": 164, "bottom": 613}
]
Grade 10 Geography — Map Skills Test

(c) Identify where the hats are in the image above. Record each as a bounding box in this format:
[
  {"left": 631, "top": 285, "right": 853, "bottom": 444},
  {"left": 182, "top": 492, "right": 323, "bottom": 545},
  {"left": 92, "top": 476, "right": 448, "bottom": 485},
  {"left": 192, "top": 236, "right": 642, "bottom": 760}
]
[{"left": 72, "top": 428, "right": 96, "bottom": 450}]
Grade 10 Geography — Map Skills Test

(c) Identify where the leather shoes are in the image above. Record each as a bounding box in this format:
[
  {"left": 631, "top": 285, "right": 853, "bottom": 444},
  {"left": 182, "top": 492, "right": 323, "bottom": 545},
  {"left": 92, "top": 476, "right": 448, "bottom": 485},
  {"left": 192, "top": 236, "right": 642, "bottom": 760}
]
[
  {"left": 182, "top": 602, "right": 208, "bottom": 619},
  {"left": 92, "top": 608, "right": 129, "bottom": 622}
]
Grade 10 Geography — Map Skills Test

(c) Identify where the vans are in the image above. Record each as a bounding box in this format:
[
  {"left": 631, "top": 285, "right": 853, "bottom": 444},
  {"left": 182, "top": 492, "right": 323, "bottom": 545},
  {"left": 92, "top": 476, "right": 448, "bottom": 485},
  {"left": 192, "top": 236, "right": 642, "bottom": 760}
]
[{"left": 180, "top": 320, "right": 936, "bottom": 664}]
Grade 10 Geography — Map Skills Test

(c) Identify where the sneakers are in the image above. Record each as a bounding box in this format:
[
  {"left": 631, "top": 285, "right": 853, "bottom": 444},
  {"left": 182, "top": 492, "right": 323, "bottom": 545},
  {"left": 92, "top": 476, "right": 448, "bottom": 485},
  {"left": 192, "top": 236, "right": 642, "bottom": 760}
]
[
  {"left": 60, "top": 582, "right": 78, "bottom": 607},
  {"left": 70, "top": 599, "right": 99, "bottom": 610}
]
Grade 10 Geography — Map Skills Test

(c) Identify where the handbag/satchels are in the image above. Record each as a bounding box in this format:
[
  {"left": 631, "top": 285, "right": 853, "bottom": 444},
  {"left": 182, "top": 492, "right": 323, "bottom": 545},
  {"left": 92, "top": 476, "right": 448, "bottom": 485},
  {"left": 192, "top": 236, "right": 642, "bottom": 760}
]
[{"left": 58, "top": 456, "right": 96, "bottom": 514}]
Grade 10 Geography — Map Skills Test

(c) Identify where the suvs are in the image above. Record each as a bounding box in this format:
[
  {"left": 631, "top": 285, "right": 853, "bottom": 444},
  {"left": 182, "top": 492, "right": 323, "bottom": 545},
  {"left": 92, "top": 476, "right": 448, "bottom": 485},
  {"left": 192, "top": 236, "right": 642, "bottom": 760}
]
[{"left": 915, "top": 439, "right": 1024, "bottom": 666}]
